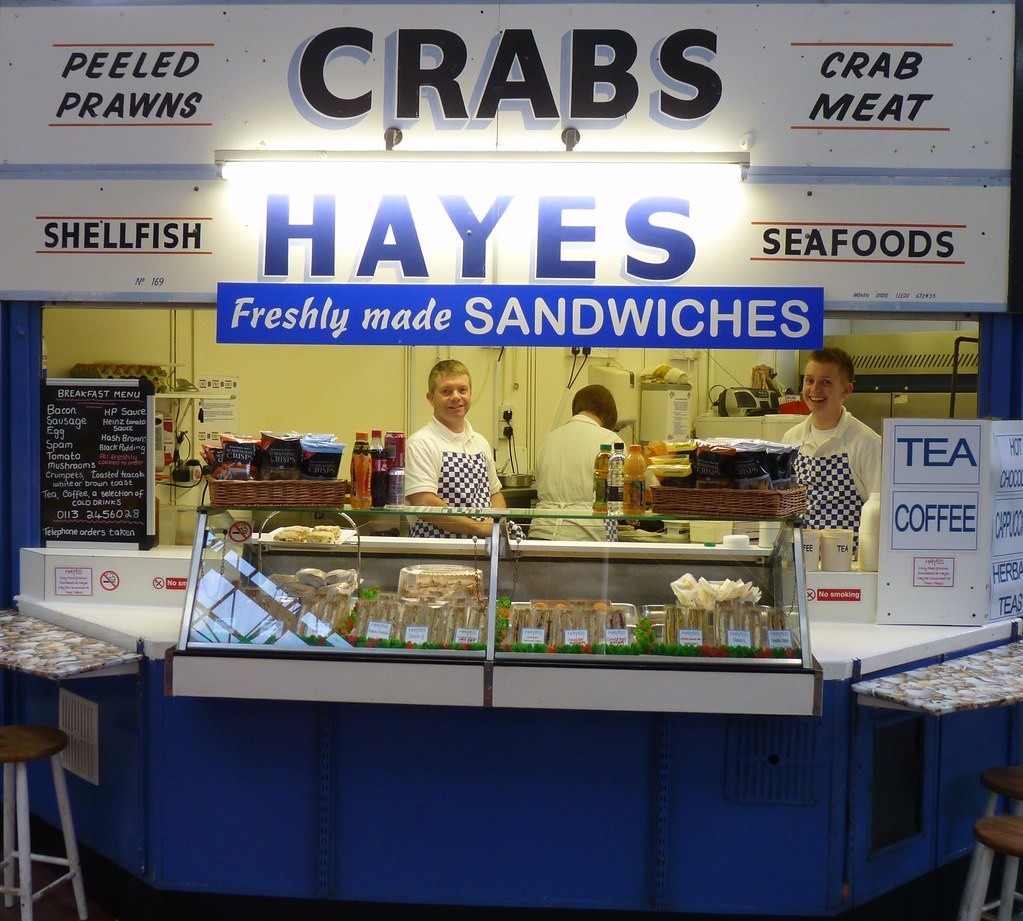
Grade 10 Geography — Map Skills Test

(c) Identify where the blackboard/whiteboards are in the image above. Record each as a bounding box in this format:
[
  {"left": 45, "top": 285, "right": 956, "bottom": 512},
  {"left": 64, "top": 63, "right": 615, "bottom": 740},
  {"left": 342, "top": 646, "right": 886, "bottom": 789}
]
[{"left": 40, "top": 377, "right": 155, "bottom": 551}]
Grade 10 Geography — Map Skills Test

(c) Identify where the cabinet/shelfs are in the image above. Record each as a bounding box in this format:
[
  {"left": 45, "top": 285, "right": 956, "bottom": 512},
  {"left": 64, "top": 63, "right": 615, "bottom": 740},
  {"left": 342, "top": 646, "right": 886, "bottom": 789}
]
[{"left": 163, "top": 502, "right": 825, "bottom": 718}]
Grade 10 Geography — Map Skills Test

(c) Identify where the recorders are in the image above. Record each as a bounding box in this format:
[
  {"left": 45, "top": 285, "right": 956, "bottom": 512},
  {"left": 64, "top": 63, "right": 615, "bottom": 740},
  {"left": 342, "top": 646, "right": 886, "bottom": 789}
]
[{"left": 705, "top": 350, "right": 781, "bottom": 417}]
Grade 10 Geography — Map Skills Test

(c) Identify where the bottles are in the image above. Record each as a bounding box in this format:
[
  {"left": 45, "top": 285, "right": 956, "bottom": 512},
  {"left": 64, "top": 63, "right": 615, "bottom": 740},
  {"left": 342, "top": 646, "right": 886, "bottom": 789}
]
[
  {"left": 369, "top": 430, "right": 389, "bottom": 509},
  {"left": 858, "top": 493, "right": 879, "bottom": 571},
  {"left": 388, "top": 466, "right": 406, "bottom": 507},
  {"left": 621, "top": 444, "right": 647, "bottom": 515},
  {"left": 385, "top": 430, "right": 405, "bottom": 468},
  {"left": 591, "top": 444, "right": 613, "bottom": 512},
  {"left": 606, "top": 442, "right": 628, "bottom": 512},
  {"left": 350, "top": 432, "right": 373, "bottom": 509}
]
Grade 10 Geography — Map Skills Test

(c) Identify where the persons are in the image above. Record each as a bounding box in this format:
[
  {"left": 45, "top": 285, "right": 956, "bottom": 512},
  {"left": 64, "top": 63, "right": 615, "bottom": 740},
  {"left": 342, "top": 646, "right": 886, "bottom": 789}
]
[
  {"left": 781, "top": 348, "right": 881, "bottom": 562},
  {"left": 526, "top": 384, "right": 640, "bottom": 543},
  {"left": 405, "top": 359, "right": 511, "bottom": 539}
]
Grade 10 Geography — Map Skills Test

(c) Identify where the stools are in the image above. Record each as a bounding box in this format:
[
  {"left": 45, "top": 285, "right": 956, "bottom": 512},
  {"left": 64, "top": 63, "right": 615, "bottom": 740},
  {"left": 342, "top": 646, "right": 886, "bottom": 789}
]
[
  {"left": 980, "top": 764, "right": 1022, "bottom": 822},
  {"left": 0, "top": 726, "right": 90, "bottom": 921},
  {"left": 954, "top": 815, "right": 1023, "bottom": 921}
]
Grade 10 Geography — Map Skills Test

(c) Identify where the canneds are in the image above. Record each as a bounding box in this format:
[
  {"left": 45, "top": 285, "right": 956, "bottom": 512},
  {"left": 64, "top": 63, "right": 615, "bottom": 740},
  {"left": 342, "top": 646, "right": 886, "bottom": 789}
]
[
  {"left": 383, "top": 431, "right": 404, "bottom": 470},
  {"left": 388, "top": 467, "right": 404, "bottom": 507}
]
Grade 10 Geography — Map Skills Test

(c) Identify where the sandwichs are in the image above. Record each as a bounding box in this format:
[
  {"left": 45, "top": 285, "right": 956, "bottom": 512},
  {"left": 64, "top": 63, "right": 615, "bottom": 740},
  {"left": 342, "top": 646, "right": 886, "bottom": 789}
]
[{"left": 258, "top": 564, "right": 787, "bottom": 649}]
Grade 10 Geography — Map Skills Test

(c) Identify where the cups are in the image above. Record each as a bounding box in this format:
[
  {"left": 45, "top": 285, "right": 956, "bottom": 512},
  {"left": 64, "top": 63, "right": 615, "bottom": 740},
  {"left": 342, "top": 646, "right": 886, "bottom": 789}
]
[
  {"left": 785, "top": 528, "right": 820, "bottom": 570},
  {"left": 820, "top": 528, "right": 854, "bottom": 572}
]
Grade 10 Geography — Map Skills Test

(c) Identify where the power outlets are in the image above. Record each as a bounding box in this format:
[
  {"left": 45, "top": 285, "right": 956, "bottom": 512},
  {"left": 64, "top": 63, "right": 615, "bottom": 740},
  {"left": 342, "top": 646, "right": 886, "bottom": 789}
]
[
  {"left": 499, "top": 422, "right": 514, "bottom": 438},
  {"left": 499, "top": 405, "right": 513, "bottom": 421},
  {"left": 671, "top": 349, "right": 702, "bottom": 361},
  {"left": 174, "top": 460, "right": 203, "bottom": 481},
  {"left": 565, "top": 347, "right": 618, "bottom": 359}
]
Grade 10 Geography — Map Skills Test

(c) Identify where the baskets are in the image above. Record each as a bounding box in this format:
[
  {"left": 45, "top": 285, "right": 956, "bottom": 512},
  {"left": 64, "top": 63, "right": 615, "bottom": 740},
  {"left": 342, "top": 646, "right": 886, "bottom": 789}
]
[
  {"left": 649, "top": 485, "right": 807, "bottom": 519},
  {"left": 205, "top": 473, "right": 347, "bottom": 508}
]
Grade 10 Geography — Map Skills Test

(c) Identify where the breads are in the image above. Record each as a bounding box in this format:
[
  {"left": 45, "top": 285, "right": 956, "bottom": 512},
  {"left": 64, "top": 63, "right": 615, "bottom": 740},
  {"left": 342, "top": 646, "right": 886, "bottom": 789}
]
[{"left": 272, "top": 525, "right": 342, "bottom": 546}]
